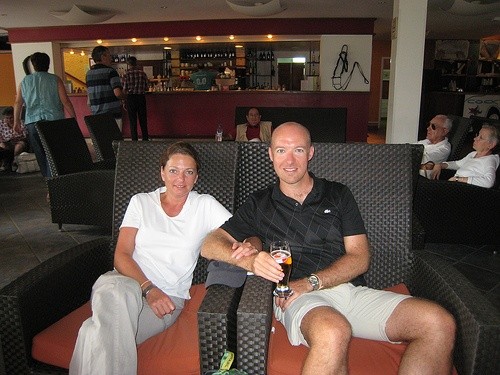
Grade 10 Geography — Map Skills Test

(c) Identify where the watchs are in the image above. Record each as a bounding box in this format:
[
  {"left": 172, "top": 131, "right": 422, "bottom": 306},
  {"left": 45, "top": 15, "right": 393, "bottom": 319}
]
[{"left": 307, "top": 273, "right": 319, "bottom": 291}]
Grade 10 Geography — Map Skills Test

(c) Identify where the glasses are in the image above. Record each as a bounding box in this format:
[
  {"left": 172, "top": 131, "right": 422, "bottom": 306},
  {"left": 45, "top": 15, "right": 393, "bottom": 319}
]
[{"left": 428, "top": 122, "right": 444, "bottom": 131}]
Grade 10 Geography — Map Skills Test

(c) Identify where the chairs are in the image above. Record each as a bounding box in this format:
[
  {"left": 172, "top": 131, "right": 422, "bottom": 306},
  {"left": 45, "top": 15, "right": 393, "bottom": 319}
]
[
  {"left": 417, "top": 119, "right": 500, "bottom": 248},
  {"left": 0, "top": 133, "right": 231, "bottom": 375},
  {"left": 440, "top": 115, "right": 471, "bottom": 162},
  {"left": 232, "top": 133, "right": 500, "bottom": 375},
  {"left": 79, "top": 103, "right": 121, "bottom": 160},
  {"left": 31, "top": 110, "right": 112, "bottom": 232}
]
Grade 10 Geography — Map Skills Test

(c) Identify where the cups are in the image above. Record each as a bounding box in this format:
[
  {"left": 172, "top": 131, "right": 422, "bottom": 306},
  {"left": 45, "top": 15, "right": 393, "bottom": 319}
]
[{"left": 270, "top": 240, "right": 294, "bottom": 298}]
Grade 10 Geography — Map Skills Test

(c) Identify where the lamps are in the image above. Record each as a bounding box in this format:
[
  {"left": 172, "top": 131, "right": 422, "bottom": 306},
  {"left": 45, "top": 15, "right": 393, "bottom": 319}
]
[
  {"left": 79, "top": 50, "right": 85, "bottom": 56},
  {"left": 69, "top": 49, "right": 75, "bottom": 55}
]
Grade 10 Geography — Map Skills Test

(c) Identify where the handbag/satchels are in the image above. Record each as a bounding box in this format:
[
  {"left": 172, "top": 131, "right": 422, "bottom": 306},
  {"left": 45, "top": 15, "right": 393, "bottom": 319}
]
[{"left": 16, "top": 152, "right": 41, "bottom": 173}]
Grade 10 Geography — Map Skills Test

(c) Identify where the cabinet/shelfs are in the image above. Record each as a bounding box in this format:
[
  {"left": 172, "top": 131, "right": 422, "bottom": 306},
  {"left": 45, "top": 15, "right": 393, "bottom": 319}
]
[{"left": 169, "top": 47, "right": 320, "bottom": 88}]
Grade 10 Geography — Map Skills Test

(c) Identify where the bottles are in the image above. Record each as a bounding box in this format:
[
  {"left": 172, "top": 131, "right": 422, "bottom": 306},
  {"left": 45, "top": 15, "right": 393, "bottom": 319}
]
[
  {"left": 113, "top": 54, "right": 127, "bottom": 78},
  {"left": 247, "top": 48, "right": 257, "bottom": 74},
  {"left": 149, "top": 73, "right": 178, "bottom": 92},
  {"left": 180, "top": 47, "right": 235, "bottom": 82},
  {"left": 271, "top": 52, "right": 274, "bottom": 60},
  {"left": 312, "top": 53, "right": 315, "bottom": 62},
  {"left": 216, "top": 128, "right": 223, "bottom": 142},
  {"left": 312, "top": 64, "right": 315, "bottom": 74},
  {"left": 267, "top": 52, "right": 270, "bottom": 60},
  {"left": 260, "top": 52, "right": 266, "bottom": 60},
  {"left": 271, "top": 66, "right": 275, "bottom": 75}
]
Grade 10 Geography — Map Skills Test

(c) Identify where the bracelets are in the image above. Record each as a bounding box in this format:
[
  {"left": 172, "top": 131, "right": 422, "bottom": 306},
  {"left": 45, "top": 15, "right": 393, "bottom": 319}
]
[
  {"left": 455, "top": 175, "right": 459, "bottom": 182},
  {"left": 143, "top": 285, "right": 157, "bottom": 297},
  {"left": 140, "top": 280, "right": 150, "bottom": 287},
  {"left": 462, "top": 176, "right": 464, "bottom": 182}
]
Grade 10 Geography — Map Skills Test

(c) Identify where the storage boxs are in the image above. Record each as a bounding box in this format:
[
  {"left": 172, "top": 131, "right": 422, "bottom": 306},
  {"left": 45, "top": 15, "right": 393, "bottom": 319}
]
[{"left": 301, "top": 77, "right": 320, "bottom": 91}]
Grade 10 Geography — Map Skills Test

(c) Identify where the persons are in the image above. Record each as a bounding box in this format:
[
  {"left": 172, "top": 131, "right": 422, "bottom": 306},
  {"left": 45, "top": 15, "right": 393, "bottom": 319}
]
[
  {"left": 123, "top": 56, "right": 152, "bottom": 142},
  {"left": 409, "top": 115, "right": 453, "bottom": 181},
  {"left": 69, "top": 146, "right": 257, "bottom": 375},
  {"left": 431, "top": 125, "right": 500, "bottom": 188},
  {"left": 15, "top": 52, "right": 77, "bottom": 199},
  {"left": 0, "top": 107, "right": 28, "bottom": 172},
  {"left": 191, "top": 63, "right": 213, "bottom": 91},
  {"left": 85, "top": 46, "right": 123, "bottom": 133},
  {"left": 203, "top": 122, "right": 456, "bottom": 375},
  {"left": 235, "top": 107, "right": 271, "bottom": 144}
]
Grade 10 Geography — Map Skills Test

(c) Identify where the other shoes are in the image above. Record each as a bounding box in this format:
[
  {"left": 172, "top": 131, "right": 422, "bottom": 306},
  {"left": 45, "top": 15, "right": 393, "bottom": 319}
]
[
  {"left": 12, "top": 162, "right": 18, "bottom": 172},
  {"left": 0, "top": 164, "right": 9, "bottom": 172}
]
